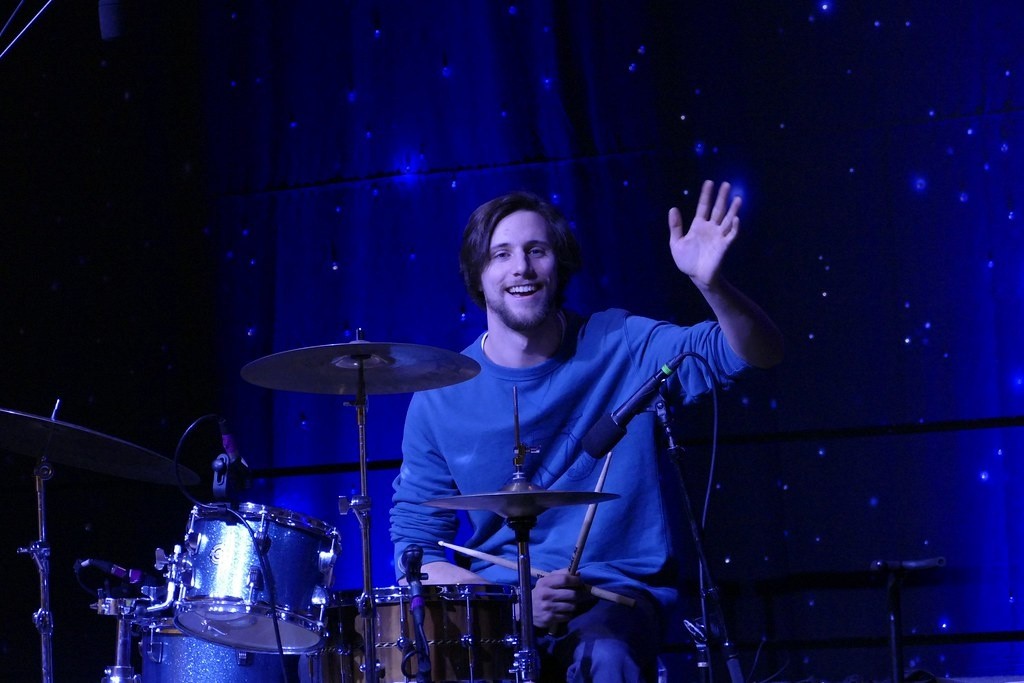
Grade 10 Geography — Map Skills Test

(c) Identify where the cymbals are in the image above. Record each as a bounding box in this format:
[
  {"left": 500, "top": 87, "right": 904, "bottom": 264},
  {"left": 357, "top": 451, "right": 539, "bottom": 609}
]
[
  {"left": 240, "top": 340, "right": 482, "bottom": 394},
  {"left": 421, "top": 492, "right": 621, "bottom": 516},
  {"left": 0, "top": 408, "right": 200, "bottom": 487}
]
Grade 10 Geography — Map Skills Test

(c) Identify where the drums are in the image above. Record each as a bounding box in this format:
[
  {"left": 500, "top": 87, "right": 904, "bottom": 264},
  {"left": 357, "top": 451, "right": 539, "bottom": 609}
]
[
  {"left": 367, "top": 582, "right": 519, "bottom": 683},
  {"left": 141, "top": 616, "right": 288, "bottom": 682},
  {"left": 299, "top": 588, "right": 367, "bottom": 682},
  {"left": 173, "top": 500, "right": 340, "bottom": 654}
]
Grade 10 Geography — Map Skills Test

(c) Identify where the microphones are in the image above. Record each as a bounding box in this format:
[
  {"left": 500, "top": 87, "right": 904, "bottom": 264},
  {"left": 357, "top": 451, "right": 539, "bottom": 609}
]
[
  {"left": 398, "top": 545, "right": 423, "bottom": 574},
  {"left": 581, "top": 355, "right": 689, "bottom": 460},
  {"left": 221, "top": 418, "right": 247, "bottom": 492},
  {"left": 89, "top": 560, "right": 157, "bottom": 590}
]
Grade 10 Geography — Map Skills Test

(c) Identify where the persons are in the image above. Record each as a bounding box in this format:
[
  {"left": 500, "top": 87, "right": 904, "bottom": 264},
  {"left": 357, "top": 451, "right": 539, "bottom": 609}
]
[{"left": 388, "top": 180, "right": 785, "bottom": 683}]
